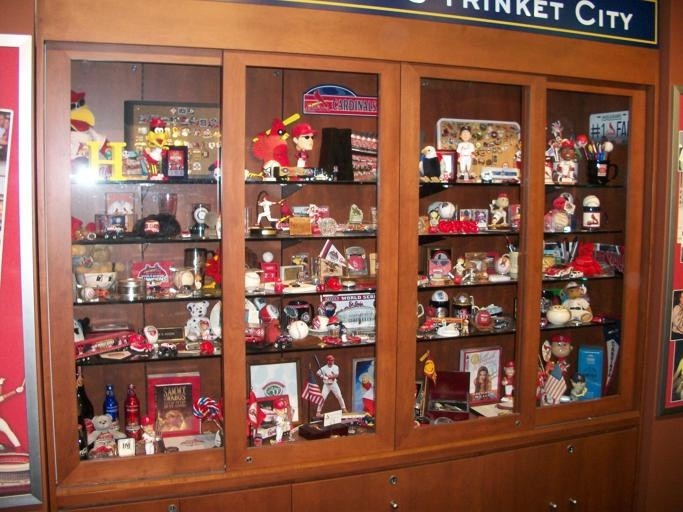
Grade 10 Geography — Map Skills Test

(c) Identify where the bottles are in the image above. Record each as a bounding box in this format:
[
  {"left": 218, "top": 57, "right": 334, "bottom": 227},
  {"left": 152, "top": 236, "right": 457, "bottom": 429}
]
[
  {"left": 102, "top": 384, "right": 119, "bottom": 433},
  {"left": 122, "top": 384, "right": 141, "bottom": 441},
  {"left": 72, "top": 365, "right": 94, "bottom": 461}
]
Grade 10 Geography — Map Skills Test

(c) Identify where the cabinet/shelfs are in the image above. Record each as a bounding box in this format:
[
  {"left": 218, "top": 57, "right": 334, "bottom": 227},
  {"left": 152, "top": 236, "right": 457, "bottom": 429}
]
[{"left": 32, "top": 1, "right": 683, "bottom": 512}]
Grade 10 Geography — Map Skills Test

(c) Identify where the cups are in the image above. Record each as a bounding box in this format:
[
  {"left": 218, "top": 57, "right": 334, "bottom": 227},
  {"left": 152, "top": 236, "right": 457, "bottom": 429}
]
[
  {"left": 553, "top": 161, "right": 579, "bottom": 185},
  {"left": 453, "top": 304, "right": 480, "bottom": 326},
  {"left": 587, "top": 158, "right": 617, "bottom": 186},
  {"left": 429, "top": 300, "right": 450, "bottom": 318},
  {"left": 159, "top": 192, "right": 177, "bottom": 215},
  {"left": 501, "top": 251, "right": 519, "bottom": 274},
  {"left": 182, "top": 247, "right": 216, "bottom": 291},
  {"left": 582, "top": 206, "right": 609, "bottom": 229}
]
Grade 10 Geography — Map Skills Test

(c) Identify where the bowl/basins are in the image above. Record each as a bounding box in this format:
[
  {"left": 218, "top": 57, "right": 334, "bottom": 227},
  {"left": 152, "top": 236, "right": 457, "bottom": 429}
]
[{"left": 78, "top": 272, "right": 116, "bottom": 290}]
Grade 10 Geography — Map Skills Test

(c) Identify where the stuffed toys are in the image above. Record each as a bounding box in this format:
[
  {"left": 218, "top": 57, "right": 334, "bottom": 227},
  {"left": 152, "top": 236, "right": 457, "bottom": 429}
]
[
  {"left": 88, "top": 414, "right": 127, "bottom": 457},
  {"left": 73, "top": 248, "right": 124, "bottom": 300}
]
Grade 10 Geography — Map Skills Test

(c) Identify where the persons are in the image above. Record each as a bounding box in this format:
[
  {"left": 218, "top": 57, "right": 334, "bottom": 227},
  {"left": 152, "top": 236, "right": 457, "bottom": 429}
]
[
  {"left": 254, "top": 195, "right": 280, "bottom": 227},
  {"left": 316, "top": 354, "right": 348, "bottom": 419},
  {"left": 290, "top": 123, "right": 320, "bottom": 168},
  {"left": 361, "top": 374, "right": 375, "bottom": 416}
]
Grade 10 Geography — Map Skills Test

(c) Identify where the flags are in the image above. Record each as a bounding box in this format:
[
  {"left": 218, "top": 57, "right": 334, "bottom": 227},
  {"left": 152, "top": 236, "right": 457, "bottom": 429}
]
[{"left": 300, "top": 367, "right": 327, "bottom": 410}]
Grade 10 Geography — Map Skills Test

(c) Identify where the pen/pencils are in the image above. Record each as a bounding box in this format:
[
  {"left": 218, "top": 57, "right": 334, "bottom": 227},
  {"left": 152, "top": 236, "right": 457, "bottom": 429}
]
[
  {"left": 505, "top": 234, "right": 520, "bottom": 252},
  {"left": 557, "top": 236, "right": 579, "bottom": 265},
  {"left": 576, "top": 134, "right": 613, "bottom": 161}
]
